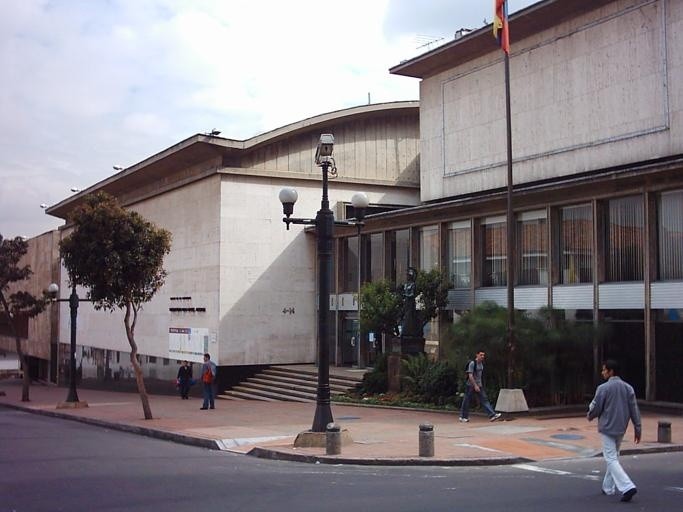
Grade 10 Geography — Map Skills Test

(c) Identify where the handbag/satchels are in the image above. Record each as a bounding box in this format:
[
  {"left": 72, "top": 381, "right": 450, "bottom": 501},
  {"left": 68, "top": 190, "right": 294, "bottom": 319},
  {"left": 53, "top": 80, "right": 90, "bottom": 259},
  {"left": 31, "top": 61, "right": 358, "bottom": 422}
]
[{"left": 202, "top": 368, "right": 214, "bottom": 384}]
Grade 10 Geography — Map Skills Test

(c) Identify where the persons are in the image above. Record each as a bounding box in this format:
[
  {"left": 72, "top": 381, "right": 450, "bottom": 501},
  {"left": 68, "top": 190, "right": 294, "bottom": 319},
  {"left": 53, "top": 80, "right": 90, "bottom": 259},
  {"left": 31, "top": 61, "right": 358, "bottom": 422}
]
[
  {"left": 585, "top": 357, "right": 642, "bottom": 502},
  {"left": 175, "top": 360, "right": 192, "bottom": 399},
  {"left": 457, "top": 350, "right": 501, "bottom": 422},
  {"left": 198, "top": 353, "right": 217, "bottom": 410}
]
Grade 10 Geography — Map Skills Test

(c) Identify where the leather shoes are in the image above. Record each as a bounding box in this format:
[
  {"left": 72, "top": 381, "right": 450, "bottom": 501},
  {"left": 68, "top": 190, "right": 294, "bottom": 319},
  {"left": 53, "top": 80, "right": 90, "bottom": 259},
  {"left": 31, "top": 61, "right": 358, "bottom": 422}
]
[{"left": 620, "top": 487, "right": 637, "bottom": 503}]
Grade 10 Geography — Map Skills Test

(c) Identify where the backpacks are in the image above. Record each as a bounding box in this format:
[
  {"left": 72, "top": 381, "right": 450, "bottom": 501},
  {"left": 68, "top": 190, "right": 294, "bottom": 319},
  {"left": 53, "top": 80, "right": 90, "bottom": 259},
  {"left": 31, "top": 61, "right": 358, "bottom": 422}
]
[{"left": 464, "top": 359, "right": 477, "bottom": 381}]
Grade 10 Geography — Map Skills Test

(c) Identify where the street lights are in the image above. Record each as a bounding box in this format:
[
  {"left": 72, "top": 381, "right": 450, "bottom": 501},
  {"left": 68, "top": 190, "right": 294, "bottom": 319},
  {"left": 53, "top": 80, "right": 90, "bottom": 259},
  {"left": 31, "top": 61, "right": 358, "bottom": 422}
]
[
  {"left": 277, "top": 188, "right": 368, "bottom": 445},
  {"left": 47, "top": 282, "right": 89, "bottom": 409}
]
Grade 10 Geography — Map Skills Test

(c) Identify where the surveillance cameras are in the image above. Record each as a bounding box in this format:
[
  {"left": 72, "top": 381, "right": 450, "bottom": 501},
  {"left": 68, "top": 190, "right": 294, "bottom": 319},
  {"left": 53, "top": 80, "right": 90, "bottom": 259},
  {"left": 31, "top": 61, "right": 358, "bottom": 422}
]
[{"left": 313, "top": 132, "right": 337, "bottom": 168}]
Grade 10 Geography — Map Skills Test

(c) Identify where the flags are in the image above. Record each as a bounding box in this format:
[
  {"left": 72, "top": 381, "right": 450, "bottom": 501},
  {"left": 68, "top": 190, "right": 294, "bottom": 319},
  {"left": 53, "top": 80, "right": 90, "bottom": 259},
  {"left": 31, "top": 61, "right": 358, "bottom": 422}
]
[{"left": 492, "top": 0, "right": 509, "bottom": 54}]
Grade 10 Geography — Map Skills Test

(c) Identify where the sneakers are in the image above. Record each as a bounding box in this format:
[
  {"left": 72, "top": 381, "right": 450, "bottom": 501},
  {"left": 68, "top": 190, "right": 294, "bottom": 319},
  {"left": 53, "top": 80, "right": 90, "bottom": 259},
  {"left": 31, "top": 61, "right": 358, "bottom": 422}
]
[
  {"left": 490, "top": 413, "right": 501, "bottom": 422},
  {"left": 459, "top": 417, "right": 468, "bottom": 422}
]
